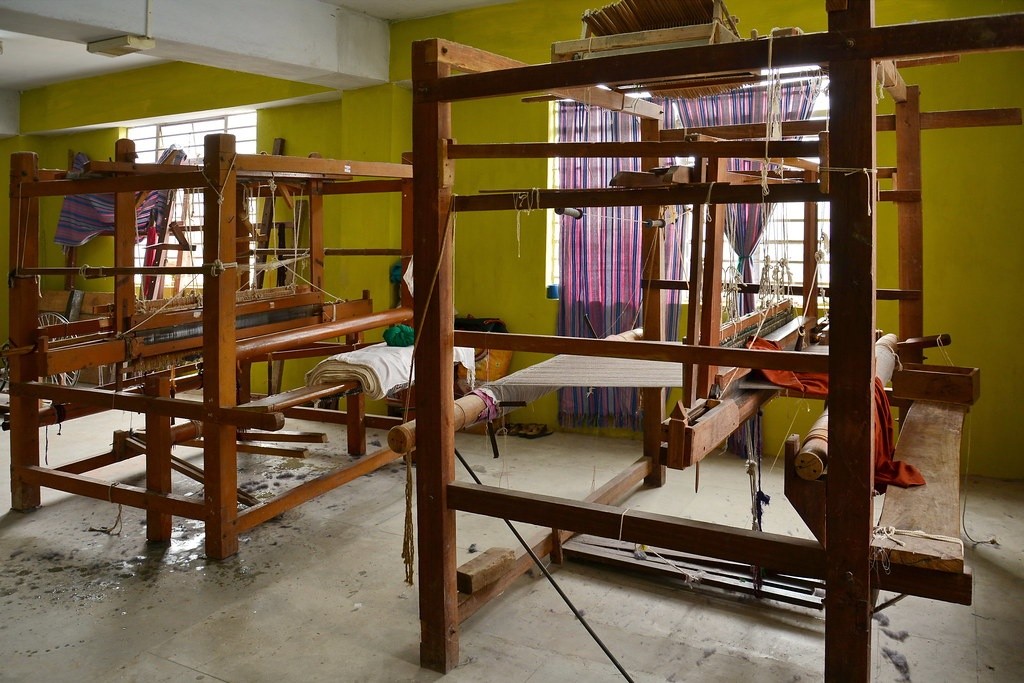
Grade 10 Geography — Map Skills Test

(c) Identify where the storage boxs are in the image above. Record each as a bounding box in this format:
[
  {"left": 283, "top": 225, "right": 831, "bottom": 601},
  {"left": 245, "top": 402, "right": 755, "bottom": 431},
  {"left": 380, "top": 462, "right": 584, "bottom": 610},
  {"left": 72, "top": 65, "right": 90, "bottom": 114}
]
[
  {"left": 891, "top": 361, "right": 982, "bottom": 405},
  {"left": 384, "top": 347, "right": 515, "bottom": 435}
]
